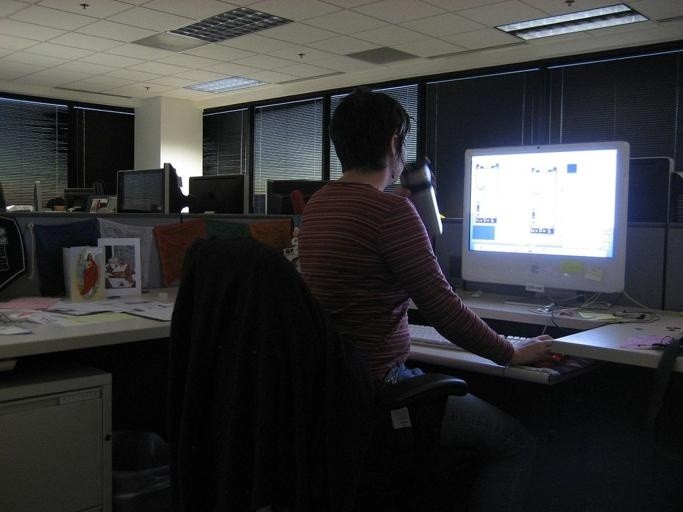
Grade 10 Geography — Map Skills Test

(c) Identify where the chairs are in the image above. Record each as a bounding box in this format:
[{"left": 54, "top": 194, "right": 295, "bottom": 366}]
[{"left": 161, "top": 234, "right": 472, "bottom": 511}]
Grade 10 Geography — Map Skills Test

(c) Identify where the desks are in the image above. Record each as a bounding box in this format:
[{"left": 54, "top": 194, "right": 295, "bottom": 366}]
[
  {"left": 404, "top": 215, "right": 682, "bottom": 384},
  {"left": 0, "top": 213, "right": 184, "bottom": 358}
]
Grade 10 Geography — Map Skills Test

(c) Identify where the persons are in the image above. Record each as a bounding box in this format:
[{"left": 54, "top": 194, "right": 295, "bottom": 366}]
[
  {"left": 296, "top": 90, "right": 559, "bottom": 512},
  {"left": 77, "top": 252, "right": 98, "bottom": 298}
]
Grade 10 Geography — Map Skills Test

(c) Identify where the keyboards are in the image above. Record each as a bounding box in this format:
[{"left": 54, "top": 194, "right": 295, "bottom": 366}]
[{"left": 408, "top": 324, "right": 530, "bottom": 358}]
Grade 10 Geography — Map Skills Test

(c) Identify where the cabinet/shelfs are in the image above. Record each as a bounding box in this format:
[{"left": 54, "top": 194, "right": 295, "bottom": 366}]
[{"left": 0, "top": 369, "right": 117, "bottom": 511}]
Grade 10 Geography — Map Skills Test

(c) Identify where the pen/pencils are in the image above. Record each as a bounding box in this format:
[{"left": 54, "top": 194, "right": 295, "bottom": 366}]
[{"left": 613, "top": 311, "right": 645, "bottom": 319}]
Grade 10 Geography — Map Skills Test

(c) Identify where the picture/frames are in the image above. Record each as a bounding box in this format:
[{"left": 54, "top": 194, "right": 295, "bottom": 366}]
[
  {"left": 88, "top": 198, "right": 109, "bottom": 214},
  {"left": 96, "top": 237, "right": 143, "bottom": 296}
]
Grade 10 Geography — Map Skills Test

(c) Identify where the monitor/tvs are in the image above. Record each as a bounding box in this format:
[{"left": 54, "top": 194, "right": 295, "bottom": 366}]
[
  {"left": 461, "top": 140, "right": 630, "bottom": 309},
  {"left": 162, "top": 164, "right": 186, "bottom": 214},
  {"left": 64, "top": 187, "right": 93, "bottom": 209},
  {"left": 264, "top": 178, "right": 327, "bottom": 215},
  {"left": 188, "top": 175, "right": 250, "bottom": 215},
  {"left": 116, "top": 169, "right": 162, "bottom": 212},
  {"left": 32, "top": 181, "right": 43, "bottom": 211}
]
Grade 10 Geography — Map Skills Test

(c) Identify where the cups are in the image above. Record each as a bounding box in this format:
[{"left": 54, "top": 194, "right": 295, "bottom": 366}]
[{"left": 398, "top": 158, "right": 444, "bottom": 237}]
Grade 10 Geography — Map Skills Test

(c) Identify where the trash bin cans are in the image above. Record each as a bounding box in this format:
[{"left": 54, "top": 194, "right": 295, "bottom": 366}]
[{"left": 112, "top": 431, "right": 176, "bottom": 512}]
